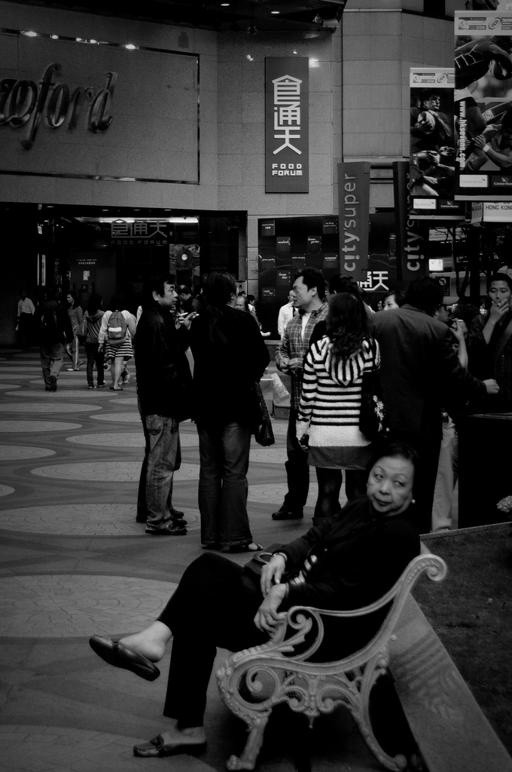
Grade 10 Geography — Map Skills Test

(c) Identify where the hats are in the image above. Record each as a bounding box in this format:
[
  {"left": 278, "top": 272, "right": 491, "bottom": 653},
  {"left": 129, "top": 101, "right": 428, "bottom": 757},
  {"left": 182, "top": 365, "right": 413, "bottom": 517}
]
[{"left": 441, "top": 295, "right": 459, "bottom": 308}]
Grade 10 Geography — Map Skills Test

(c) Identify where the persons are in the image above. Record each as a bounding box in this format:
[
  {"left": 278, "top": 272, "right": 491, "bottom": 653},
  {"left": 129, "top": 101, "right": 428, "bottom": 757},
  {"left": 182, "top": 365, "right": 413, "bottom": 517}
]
[
  {"left": 410, "top": 38, "right": 512, "bottom": 195},
  {"left": 90, "top": 438, "right": 421, "bottom": 758},
  {"left": 15, "top": 289, "right": 137, "bottom": 391},
  {"left": 131, "top": 268, "right": 511, "bottom": 556}
]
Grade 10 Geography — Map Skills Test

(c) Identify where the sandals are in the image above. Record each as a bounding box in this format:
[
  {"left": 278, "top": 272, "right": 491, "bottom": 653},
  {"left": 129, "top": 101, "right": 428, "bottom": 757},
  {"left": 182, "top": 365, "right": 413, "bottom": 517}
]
[{"left": 221, "top": 540, "right": 262, "bottom": 552}]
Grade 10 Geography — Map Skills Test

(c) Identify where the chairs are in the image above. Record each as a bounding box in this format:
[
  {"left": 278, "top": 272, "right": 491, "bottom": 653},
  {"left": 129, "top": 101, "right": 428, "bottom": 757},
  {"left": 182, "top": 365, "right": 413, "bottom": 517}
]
[{"left": 209, "top": 537, "right": 455, "bottom": 772}]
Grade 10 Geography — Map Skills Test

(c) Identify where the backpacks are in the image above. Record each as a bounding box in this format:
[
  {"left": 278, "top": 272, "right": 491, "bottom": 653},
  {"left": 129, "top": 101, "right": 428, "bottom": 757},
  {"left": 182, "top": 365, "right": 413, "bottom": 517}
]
[{"left": 108, "top": 308, "right": 125, "bottom": 342}]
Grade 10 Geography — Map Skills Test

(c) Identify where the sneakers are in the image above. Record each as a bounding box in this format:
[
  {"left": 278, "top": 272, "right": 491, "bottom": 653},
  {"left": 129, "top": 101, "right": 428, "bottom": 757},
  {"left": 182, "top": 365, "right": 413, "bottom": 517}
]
[
  {"left": 273, "top": 510, "right": 303, "bottom": 520},
  {"left": 145, "top": 510, "right": 188, "bottom": 534}
]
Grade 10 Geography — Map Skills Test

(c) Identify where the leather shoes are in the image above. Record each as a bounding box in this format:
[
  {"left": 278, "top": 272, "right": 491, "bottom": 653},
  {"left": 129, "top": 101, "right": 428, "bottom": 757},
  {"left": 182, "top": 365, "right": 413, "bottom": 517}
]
[
  {"left": 91, "top": 635, "right": 161, "bottom": 682},
  {"left": 134, "top": 734, "right": 208, "bottom": 757}
]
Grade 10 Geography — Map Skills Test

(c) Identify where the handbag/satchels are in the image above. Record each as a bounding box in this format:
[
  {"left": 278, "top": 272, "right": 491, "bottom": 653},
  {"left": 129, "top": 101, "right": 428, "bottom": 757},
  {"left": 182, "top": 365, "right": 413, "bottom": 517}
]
[
  {"left": 245, "top": 544, "right": 285, "bottom": 575},
  {"left": 360, "top": 370, "right": 392, "bottom": 440}
]
